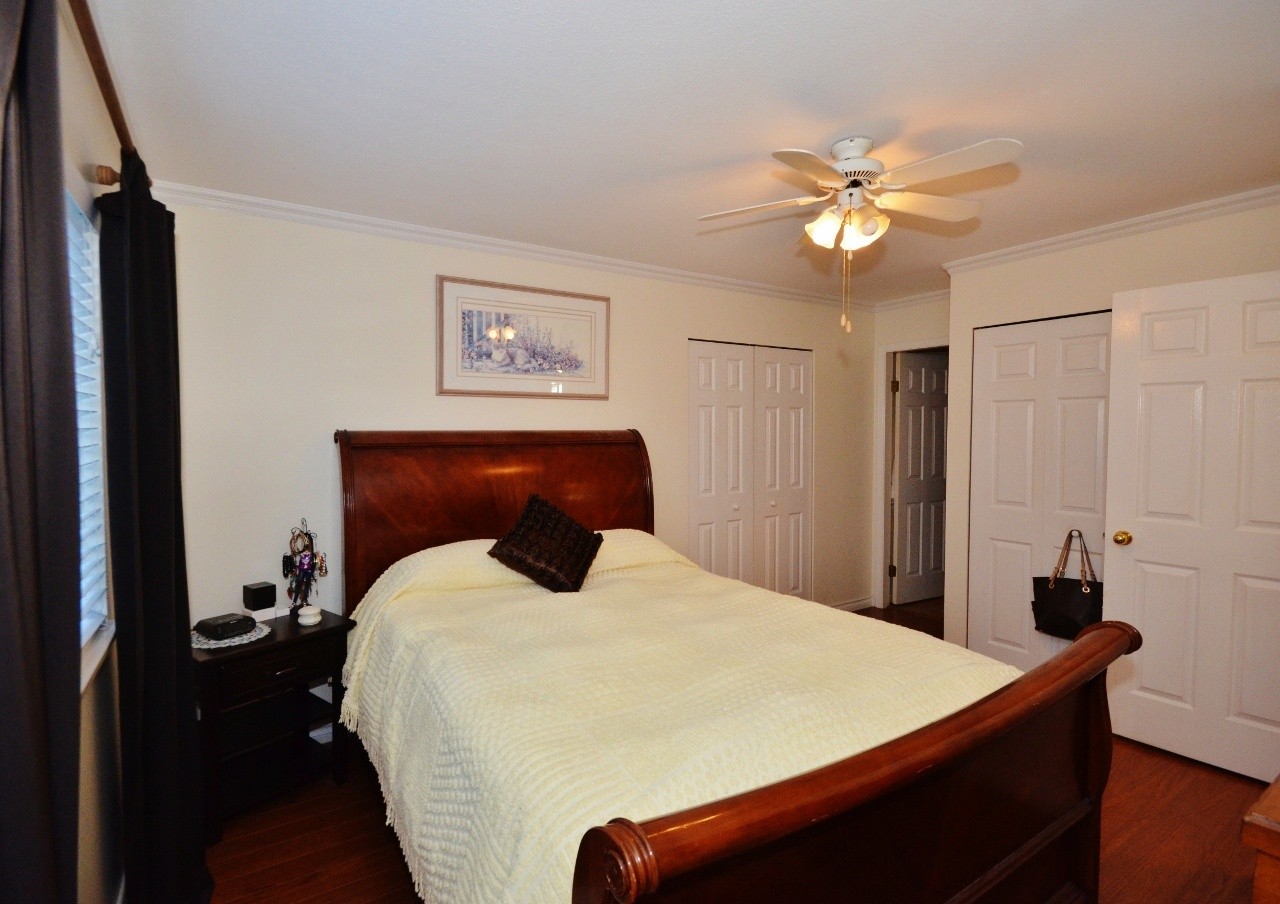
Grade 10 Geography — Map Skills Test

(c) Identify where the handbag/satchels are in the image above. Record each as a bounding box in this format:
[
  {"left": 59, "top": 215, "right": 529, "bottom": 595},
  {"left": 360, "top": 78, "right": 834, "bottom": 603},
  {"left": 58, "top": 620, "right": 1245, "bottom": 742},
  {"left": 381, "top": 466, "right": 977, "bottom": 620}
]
[{"left": 1031, "top": 528, "right": 1105, "bottom": 641}]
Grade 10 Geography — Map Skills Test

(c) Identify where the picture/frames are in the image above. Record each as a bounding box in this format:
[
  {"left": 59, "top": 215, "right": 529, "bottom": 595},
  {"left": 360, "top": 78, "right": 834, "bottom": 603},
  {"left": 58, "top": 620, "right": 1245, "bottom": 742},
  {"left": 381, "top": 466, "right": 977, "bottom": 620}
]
[{"left": 436, "top": 274, "right": 611, "bottom": 401}]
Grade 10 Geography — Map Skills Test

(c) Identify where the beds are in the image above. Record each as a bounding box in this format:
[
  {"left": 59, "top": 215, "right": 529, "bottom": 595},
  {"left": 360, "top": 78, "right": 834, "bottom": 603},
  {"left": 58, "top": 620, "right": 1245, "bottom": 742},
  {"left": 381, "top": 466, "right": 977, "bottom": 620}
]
[{"left": 332, "top": 428, "right": 1144, "bottom": 904}]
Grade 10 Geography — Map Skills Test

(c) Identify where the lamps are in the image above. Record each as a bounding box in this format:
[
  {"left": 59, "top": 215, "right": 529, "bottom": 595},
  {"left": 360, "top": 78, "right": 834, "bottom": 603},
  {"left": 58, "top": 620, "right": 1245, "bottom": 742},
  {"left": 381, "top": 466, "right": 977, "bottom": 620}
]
[{"left": 803, "top": 202, "right": 890, "bottom": 334}]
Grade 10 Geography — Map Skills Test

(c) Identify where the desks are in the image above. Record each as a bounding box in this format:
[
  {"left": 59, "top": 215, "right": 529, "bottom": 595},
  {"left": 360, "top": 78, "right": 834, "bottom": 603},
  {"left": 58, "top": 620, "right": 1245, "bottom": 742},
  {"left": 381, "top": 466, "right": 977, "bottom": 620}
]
[{"left": 1240, "top": 774, "right": 1280, "bottom": 903}]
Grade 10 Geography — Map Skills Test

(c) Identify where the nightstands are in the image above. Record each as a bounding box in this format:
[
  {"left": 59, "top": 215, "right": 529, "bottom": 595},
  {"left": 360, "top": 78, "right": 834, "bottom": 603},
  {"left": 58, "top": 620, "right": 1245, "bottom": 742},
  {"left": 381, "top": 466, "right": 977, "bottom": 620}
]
[{"left": 190, "top": 608, "right": 357, "bottom": 846}]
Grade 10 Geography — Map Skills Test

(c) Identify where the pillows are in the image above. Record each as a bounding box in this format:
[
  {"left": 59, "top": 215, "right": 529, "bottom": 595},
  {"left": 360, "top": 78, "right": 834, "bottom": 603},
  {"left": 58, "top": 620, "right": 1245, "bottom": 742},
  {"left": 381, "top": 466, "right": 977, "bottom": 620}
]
[{"left": 487, "top": 492, "right": 604, "bottom": 592}]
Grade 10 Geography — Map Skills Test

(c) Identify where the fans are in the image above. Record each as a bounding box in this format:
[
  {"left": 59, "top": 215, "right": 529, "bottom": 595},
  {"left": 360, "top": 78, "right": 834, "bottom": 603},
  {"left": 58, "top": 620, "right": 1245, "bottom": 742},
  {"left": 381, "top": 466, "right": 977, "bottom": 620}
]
[{"left": 692, "top": 137, "right": 1025, "bottom": 227}]
